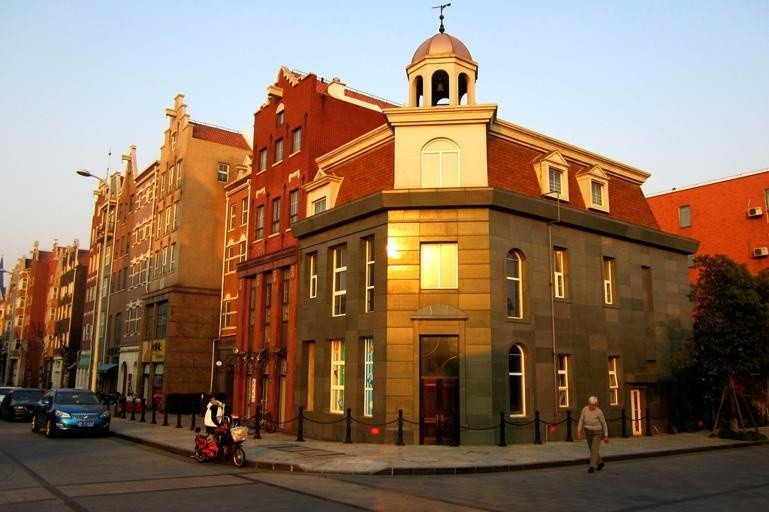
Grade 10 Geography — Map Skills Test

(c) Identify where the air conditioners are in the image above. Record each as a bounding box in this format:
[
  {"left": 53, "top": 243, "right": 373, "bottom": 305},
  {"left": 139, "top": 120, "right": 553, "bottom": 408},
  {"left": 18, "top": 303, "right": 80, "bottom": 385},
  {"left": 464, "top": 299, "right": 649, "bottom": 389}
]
[
  {"left": 753, "top": 247, "right": 768, "bottom": 257},
  {"left": 748, "top": 206, "right": 763, "bottom": 217}
]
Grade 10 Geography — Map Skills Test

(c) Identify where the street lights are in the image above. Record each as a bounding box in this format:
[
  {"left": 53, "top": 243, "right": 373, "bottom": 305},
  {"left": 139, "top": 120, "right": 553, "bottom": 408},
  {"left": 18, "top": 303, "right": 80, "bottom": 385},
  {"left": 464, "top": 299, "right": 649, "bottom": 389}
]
[
  {"left": 75, "top": 167, "right": 112, "bottom": 393},
  {"left": 0, "top": 387, "right": 111, "bottom": 437},
  {"left": 0, "top": 269, "right": 19, "bottom": 387}
]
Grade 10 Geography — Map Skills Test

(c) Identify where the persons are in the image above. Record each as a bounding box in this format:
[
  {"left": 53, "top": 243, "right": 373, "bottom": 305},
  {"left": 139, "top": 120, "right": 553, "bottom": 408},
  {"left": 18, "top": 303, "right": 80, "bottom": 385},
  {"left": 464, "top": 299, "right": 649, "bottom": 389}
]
[
  {"left": 575, "top": 394, "right": 610, "bottom": 473},
  {"left": 203, "top": 391, "right": 233, "bottom": 448}
]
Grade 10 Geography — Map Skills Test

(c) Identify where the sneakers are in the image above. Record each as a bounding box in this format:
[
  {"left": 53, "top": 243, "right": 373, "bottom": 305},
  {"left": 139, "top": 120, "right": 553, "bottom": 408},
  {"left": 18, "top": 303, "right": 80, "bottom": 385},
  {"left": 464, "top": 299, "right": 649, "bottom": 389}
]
[{"left": 588, "top": 462, "right": 606, "bottom": 474}]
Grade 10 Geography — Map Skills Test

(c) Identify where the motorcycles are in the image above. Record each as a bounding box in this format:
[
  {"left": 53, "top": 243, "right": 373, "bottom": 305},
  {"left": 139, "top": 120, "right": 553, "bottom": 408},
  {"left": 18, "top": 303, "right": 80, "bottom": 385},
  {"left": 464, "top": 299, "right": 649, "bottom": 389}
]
[{"left": 190, "top": 416, "right": 249, "bottom": 467}]
